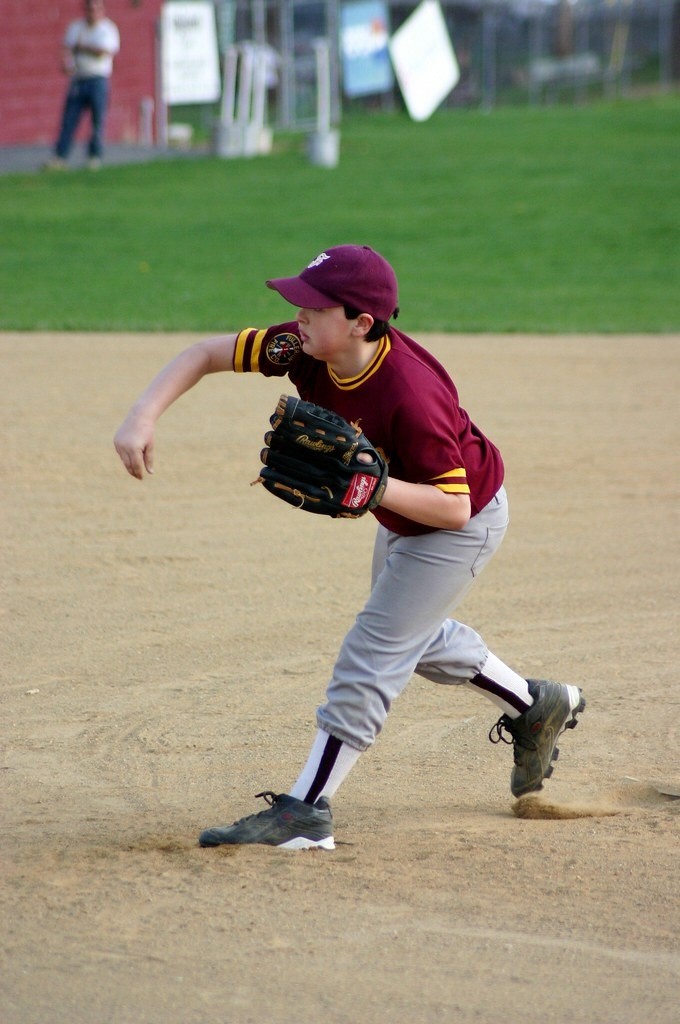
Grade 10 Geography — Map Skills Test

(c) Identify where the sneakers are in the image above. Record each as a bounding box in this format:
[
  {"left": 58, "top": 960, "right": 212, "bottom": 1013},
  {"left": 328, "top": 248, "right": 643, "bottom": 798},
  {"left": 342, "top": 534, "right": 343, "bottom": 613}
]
[
  {"left": 200, "top": 791, "right": 336, "bottom": 850},
  {"left": 489, "top": 678, "right": 586, "bottom": 799}
]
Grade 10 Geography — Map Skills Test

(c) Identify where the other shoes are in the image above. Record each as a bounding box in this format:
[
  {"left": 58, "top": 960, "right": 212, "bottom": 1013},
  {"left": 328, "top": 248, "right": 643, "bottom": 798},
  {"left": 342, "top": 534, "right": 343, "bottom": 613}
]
[
  {"left": 89, "top": 158, "right": 102, "bottom": 171},
  {"left": 43, "top": 157, "right": 68, "bottom": 169}
]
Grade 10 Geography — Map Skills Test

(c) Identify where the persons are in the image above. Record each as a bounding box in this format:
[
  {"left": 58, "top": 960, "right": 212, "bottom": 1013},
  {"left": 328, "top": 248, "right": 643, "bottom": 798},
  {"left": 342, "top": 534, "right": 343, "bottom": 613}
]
[
  {"left": 114, "top": 244, "right": 587, "bottom": 852},
  {"left": 42, "top": 0, "right": 121, "bottom": 170}
]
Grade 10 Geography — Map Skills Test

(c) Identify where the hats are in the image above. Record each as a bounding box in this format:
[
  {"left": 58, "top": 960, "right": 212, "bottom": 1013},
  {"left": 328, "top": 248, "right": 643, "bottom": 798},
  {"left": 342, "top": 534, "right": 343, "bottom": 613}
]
[{"left": 266, "top": 245, "right": 400, "bottom": 322}]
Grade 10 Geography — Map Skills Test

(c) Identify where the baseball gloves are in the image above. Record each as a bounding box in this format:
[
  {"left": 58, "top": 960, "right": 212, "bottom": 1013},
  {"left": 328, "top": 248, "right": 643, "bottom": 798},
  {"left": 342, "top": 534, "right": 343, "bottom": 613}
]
[{"left": 248, "top": 394, "right": 390, "bottom": 519}]
